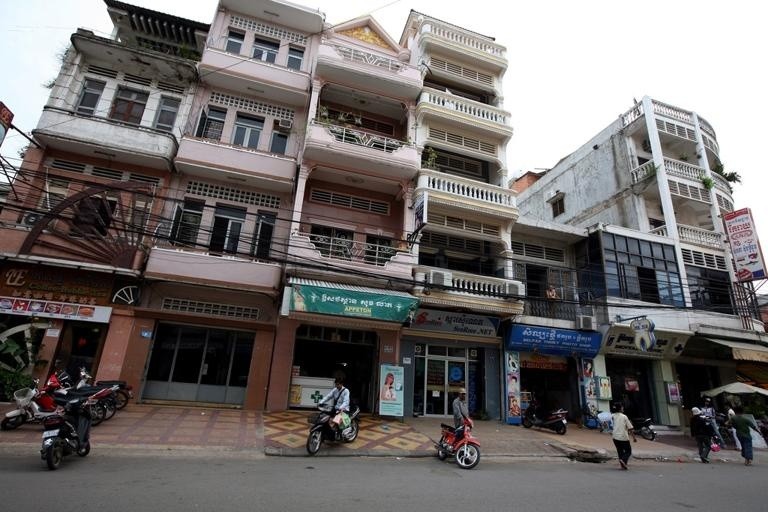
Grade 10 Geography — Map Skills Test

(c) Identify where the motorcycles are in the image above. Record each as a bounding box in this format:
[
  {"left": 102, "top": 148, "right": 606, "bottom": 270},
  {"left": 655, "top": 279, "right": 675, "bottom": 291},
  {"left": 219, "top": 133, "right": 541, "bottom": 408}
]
[
  {"left": 704, "top": 411, "right": 727, "bottom": 452},
  {"left": 435, "top": 415, "right": 482, "bottom": 470},
  {"left": 628, "top": 417, "right": 658, "bottom": 442},
  {"left": 305, "top": 401, "right": 363, "bottom": 456},
  {"left": 0, "top": 365, "right": 133, "bottom": 472},
  {"left": 521, "top": 391, "right": 569, "bottom": 436}
]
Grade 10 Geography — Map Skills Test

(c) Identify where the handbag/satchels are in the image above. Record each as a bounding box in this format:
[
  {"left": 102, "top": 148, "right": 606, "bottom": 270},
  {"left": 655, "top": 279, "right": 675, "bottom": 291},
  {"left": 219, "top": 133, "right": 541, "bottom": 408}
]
[
  {"left": 711, "top": 444, "right": 720, "bottom": 452},
  {"left": 333, "top": 412, "right": 351, "bottom": 429}
]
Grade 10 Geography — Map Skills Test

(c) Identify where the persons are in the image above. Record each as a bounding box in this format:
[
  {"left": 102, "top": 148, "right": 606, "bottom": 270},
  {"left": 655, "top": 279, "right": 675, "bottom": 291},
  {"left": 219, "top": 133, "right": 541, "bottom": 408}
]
[
  {"left": 316, "top": 375, "right": 351, "bottom": 438},
  {"left": 723, "top": 401, "right": 742, "bottom": 450},
  {"left": 508, "top": 353, "right": 518, "bottom": 373},
  {"left": 584, "top": 361, "right": 593, "bottom": 377},
  {"left": 546, "top": 285, "right": 560, "bottom": 299},
  {"left": 508, "top": 376, "right": 519, "bottom": 394},
  {"left": 724, "top": 405, "right": 762, "bottom": 465},
  {"left": 510, "top": 399, "right": 520, "bottom": 416},
  {"left": 701, "top": 397, "right": 727, "bottom": 448},
  {"left": 690, "top": 406, "right": 712, "bottom": 464},
  {"left": 380, "top": 372, "right": 397, "bottom": 401},
  {"left": 452, "top": 387, "right": 471, "bottom": 428},
  {"left": 611, "top": 402, "right": 638, "bottom": 470}
]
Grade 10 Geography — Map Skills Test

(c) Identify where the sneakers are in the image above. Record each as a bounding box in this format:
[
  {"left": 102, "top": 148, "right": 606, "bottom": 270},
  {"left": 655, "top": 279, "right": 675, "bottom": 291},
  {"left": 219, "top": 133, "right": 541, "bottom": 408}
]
[
  {"left": 699, "top": 453, "right": 709, "bottom": 463},
  {"left": 620, "top": 459, "right": 628, "bottom": 469},
  {"left": 745, "top": 458, "right": 752, "bottom": 465}
]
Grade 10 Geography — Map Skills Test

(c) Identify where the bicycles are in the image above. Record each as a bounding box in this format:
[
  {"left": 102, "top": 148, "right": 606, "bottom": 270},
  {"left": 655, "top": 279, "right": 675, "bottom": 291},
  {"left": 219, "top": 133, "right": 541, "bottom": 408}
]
[{"left": 582, "top": 402, "right": 613, "bottom": 434}]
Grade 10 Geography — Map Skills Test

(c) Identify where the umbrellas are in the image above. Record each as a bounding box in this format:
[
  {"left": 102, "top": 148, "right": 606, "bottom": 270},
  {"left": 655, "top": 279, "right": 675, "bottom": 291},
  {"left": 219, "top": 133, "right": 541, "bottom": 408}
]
[{"left": 699, "top": 381, "right": 768, "bottom": 399}]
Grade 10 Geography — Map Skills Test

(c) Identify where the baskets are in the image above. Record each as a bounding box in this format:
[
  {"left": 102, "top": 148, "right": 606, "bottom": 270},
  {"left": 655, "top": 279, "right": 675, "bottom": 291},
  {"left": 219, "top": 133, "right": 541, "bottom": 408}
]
[
  {"left": 14, "top": 387, "right": 33, "bottom": 407},
  {"left": 308, "top": 413, "right": 320, "bottom": 424}
]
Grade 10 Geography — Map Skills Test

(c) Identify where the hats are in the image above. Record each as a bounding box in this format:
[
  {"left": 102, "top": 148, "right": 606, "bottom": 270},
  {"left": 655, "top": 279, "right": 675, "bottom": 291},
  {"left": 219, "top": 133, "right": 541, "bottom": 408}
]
[
  {"left": 459, "top": 388, "right": 466, "bottom": 393},
  {"left": 692, "top": 407, "right": 702, "bottom": 416}
]
